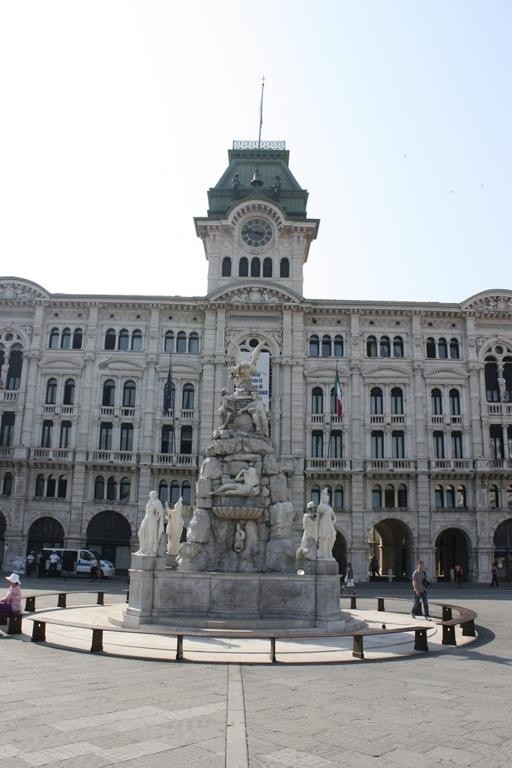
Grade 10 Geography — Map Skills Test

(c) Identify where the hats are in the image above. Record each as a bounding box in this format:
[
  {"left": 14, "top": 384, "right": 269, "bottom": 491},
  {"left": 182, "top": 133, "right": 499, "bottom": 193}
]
[{"left": 5, "top": 573, "right": 22, "bottom": 585}]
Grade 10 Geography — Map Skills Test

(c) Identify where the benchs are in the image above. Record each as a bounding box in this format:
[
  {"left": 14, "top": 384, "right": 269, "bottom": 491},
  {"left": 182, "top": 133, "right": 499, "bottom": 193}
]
[
  {"left": 27, "top": 616, "right": 432, "bottom": 662},
  {"left": 376, "top": 596, "right": 478, "bottom": 647},
  {"left": 341, "top": 592, "right": 358, "bottom": 610},
  {"left": 2, "top": 588, "right": 108, "bottom": 634}
]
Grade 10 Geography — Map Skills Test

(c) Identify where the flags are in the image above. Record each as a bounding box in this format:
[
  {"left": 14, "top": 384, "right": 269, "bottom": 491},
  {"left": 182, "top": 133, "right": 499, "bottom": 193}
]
[{"left": 335, "top": 366, "right": 343, "bottom": 416}]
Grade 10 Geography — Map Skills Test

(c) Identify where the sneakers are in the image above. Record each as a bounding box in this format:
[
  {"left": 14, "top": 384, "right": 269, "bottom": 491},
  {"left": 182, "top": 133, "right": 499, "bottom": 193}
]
[
  {"left": 425, "top": 616, "right": 431, "bottom": 621},
  {"left": 410, "top": 611, "right": 416, "bottom": 619}
]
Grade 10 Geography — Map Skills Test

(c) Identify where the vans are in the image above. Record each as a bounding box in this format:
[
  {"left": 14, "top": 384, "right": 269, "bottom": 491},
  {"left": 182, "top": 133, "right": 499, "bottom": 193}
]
[{"left": 37, "top": 545, "right": 117, "bottom": 578}]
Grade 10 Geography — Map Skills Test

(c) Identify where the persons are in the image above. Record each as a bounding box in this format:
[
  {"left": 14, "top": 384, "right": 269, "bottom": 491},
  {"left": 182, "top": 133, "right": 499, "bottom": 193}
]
[
  {"left": 237, "top": 392, "right": 270, "bottom": 438},
  {"left": 453, "top": 563, "right": 464, "bottom": 588},
  {"left": 300, "top": 501, "right": 318, "bottom": 560},
  {"left": 165, "top": 496, "right": 185, "bottom": 556},
  {"left": 491, "top": 563, "right": 499, "bottom": 587},
  {"left": 411, "top": 560, "right": 432, "bottom": 621},
  {"left": 0, "top": 574, "right": 21, "bottom": 625},
  {"left": 88, "top": 557, "right": 102, "bottom": 582},
  {"left": 216, "top": 387, "right": 235, "bottom": 429},
  {"left": 343, "top": 562, "right": 356, "bottom": 595},
  {"left": 316, "top": 495, "right": 338, "bottom": 559},
  {"left": 261, "top": 289, "right": 279, "bottom": 302},
  {"left": 138, "top": 491, "right": 165, "bottom": 554},
  {"left": 27, "top": 550, "right": 62, "bottom": 578},
  {"left": 230, "top": 290, "right": 250, "bottom": 303},
  {"left": 209, "top": 466, "right": 260, "bottom": 497},
  {"left": 370, "top": 555, "right": 381, "bottom": 578}
]
